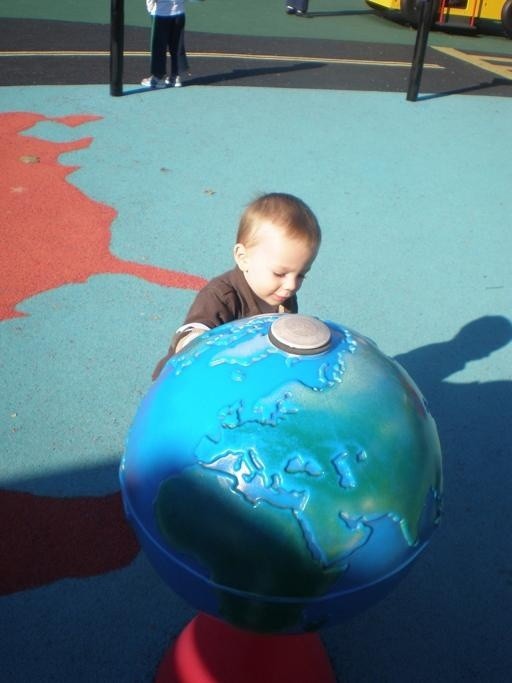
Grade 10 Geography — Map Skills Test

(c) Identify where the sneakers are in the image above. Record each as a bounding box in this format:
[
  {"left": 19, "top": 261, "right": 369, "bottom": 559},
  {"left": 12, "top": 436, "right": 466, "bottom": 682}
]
[
  {"left": 139, "top": 74, "right": 166, "bottom": 88},
  {"left": 286, "top": 6, "right": 308, "bottom": 16},
  {"left": 165, "top": 75, "right": 182, "bottom": 87}
]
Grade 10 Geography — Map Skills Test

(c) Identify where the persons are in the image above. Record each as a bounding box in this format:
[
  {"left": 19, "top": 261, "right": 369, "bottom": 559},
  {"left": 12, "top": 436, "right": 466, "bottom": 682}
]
[
  {"left": 139, "top": 0, "right": 189, "bottom": 88},
  {"left": 285, "top": 0, "right": 309, "bottom": 17},
  {"left": 150, "top": 193, "right": 323, "bottom": 381}
]
